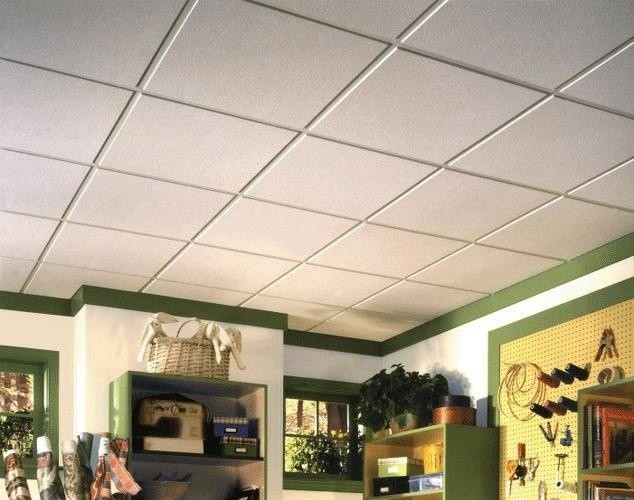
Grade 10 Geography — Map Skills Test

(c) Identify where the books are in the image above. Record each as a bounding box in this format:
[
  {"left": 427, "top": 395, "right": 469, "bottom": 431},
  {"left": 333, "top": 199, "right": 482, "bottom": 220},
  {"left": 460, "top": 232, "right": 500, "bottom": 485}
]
[
  {"left": 582, "top": 392, "right": 634, "bottom": 467},
  {"left": 582, "top": 480, "right": 634, "bottom": 500}
]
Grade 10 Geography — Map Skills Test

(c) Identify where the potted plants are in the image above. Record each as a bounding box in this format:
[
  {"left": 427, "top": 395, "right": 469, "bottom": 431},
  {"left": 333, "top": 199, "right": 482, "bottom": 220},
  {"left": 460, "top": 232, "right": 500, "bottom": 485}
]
[{"left": 357, "top": 363, "right": 449, "bottom": 433}]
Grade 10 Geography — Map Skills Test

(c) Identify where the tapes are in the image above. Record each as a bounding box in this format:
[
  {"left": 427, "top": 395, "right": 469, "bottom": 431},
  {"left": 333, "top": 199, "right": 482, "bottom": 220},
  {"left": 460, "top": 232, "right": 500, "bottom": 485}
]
[{"left": 597, "top": 367, "right": 621, "bottom": 386}]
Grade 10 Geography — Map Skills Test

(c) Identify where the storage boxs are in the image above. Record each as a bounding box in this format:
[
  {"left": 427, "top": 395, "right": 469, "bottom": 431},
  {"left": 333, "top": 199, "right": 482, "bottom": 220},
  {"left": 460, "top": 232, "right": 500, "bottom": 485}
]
[
  {"left": 373, "top": 477, "right": 409, "bottom": 496},
  {"left": 431, "top": 406, "right": 477, "bottom": 425},
  {"left": 377, "top": 457, "right": 424, "bottom": 477},
  {"left": 439, "top": 395, "right": 470, "bottom": 407},
  {"left": 219, "top": 437, "right": 259, "bottom": 458},
  {"left": 209, "top": 416, "right": 258, "bottom": 438}
]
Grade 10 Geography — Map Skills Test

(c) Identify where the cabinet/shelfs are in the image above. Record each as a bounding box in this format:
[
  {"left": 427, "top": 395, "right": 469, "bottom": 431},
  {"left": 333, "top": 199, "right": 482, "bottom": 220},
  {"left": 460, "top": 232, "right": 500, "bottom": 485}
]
[
  {"left": 109, "top": 370, "right": 267, "bottom": 500},
  {"left": 577, "top": 376, "right": 634, "bottom": 500},
  {"left": 363, "top": 425, "right": 499, "bottom": 500}
]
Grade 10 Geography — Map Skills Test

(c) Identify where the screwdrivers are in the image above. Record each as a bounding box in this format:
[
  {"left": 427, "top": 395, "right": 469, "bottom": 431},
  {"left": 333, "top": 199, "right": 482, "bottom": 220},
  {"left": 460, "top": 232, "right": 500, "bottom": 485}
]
[{"left": 519, "top": 443, "right": 523, "bottom": 477}]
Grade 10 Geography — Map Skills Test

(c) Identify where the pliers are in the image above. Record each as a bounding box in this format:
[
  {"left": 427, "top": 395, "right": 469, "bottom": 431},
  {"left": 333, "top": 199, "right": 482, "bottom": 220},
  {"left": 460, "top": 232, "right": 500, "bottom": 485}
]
[{"left": 594, "top": 328, "right": 616, "bottom": 361}]
